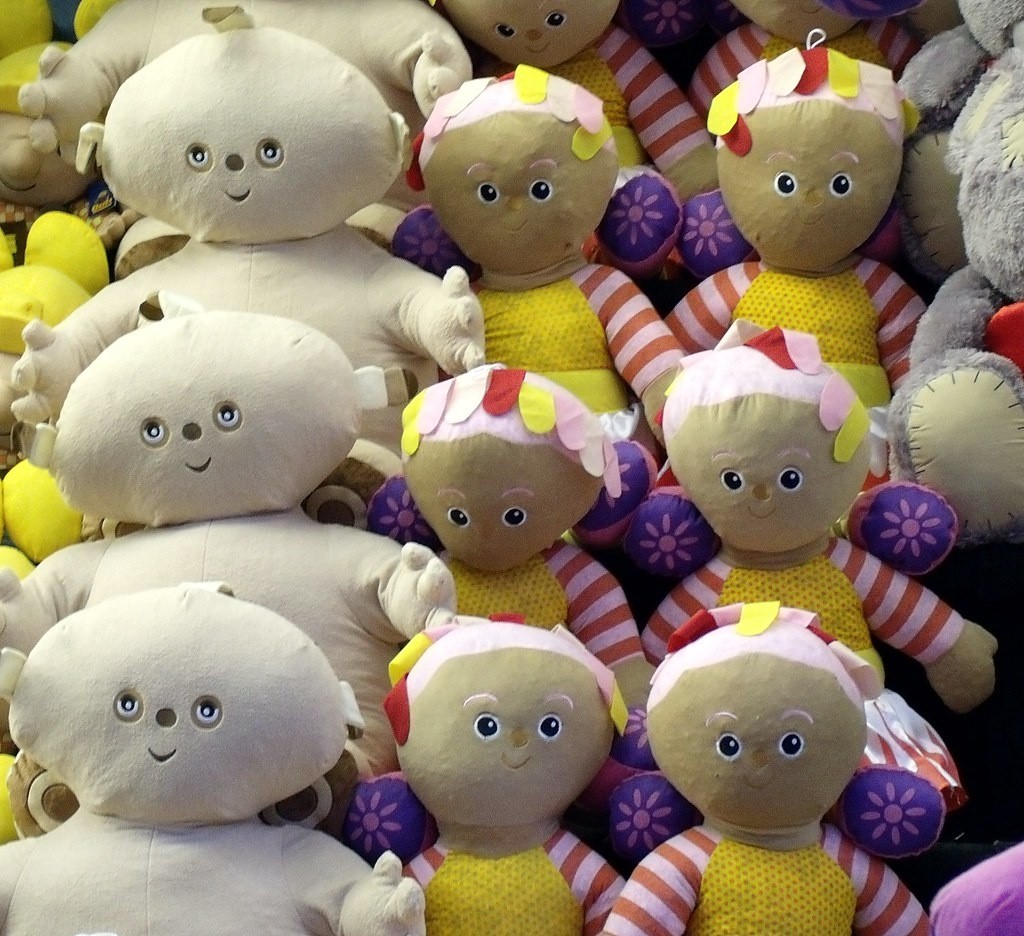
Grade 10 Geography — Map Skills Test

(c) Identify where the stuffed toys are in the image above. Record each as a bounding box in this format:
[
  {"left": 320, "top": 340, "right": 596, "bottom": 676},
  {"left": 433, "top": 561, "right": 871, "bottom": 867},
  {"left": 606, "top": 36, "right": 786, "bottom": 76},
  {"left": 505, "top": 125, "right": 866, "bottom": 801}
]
[
  {"left": 596, "top": 600, "right": 934, "bottom": 936},
  {"left": 1, "top": 581, "right": 426, "bottom": 934},
  {"left": 0, "top": 0, "right": 1024, "bottom": 935}
]
